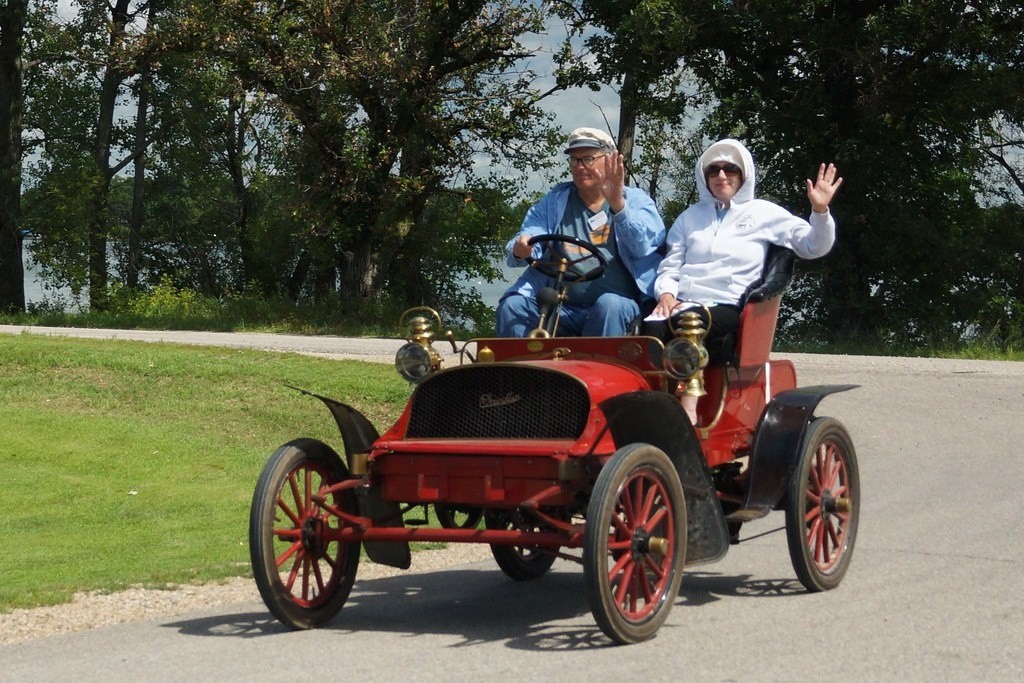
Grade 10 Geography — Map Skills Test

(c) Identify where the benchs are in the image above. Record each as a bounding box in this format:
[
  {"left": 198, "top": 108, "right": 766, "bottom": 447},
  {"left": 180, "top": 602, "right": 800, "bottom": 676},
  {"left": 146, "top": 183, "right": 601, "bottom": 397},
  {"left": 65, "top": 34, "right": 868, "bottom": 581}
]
[{"left": 637, "top": 243, "right": 795, "bottom": 369}]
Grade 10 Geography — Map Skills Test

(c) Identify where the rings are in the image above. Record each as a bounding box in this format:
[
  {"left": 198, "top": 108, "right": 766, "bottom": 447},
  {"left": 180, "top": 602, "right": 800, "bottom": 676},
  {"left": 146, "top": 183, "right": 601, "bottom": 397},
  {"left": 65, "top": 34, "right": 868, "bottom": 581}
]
[{"left": 659, "top": 306, "right": 663, "bottom": 310}]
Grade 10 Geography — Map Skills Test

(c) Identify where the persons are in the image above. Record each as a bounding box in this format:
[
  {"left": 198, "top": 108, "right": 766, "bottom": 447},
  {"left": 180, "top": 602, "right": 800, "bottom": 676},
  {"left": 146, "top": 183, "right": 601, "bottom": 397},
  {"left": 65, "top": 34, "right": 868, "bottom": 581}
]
[
  {"left": 640, "top": 138, "right": 843, "bottom": 426},
  {"left": 496, "top": 126, "right": 666, "bottom": 337}
]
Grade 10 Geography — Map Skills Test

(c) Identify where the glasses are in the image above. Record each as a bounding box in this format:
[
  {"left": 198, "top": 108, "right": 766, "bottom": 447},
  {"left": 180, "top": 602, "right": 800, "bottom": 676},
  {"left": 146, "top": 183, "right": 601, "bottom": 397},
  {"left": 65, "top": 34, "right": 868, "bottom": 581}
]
[
  {"left": 566, "top": 155, "right": 606, "bottom": 167},
  {"left": 706, "top": 163, "right": 741, "bottom": 178}
]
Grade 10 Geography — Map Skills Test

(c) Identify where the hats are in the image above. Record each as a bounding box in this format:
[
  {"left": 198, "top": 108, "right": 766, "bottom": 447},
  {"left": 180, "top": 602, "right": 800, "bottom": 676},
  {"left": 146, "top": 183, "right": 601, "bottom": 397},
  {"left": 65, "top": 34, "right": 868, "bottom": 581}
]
[{"left": 563, "top": 127, "right": 616, "bottom": 154}]
